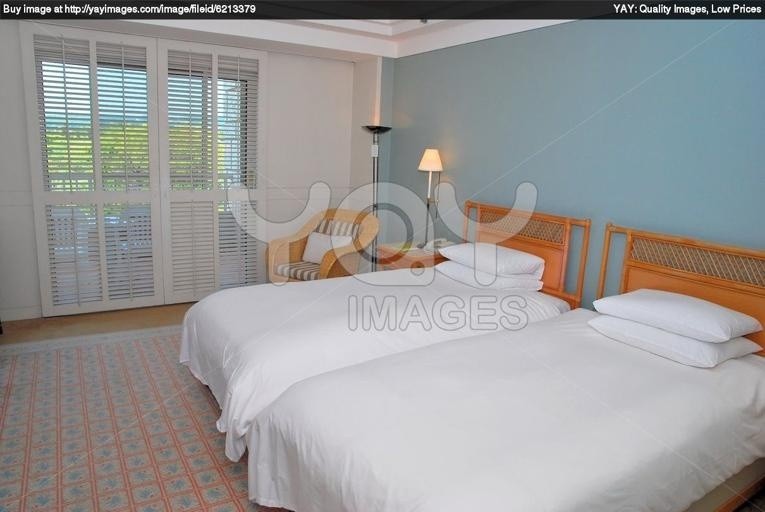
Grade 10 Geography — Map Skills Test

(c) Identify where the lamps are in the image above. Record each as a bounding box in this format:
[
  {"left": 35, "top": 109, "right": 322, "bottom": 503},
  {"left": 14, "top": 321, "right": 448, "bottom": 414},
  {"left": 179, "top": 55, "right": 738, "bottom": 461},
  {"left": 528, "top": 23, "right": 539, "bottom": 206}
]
[{"left": 416, "top": 148, "right": 443, "bottom": 248}]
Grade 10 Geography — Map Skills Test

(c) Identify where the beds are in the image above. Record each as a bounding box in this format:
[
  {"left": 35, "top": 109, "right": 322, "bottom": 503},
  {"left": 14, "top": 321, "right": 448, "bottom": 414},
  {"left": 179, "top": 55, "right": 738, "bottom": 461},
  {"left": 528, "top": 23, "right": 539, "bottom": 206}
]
[{"left": 187, "top": 202, "right": 764, "bottom": 507}]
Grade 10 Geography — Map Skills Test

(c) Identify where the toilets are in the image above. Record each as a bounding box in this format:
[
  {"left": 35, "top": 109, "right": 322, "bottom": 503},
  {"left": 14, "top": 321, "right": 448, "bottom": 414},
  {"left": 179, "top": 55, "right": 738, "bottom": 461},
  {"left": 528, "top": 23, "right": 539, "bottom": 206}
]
[{"left": 424, "top": 238, "right": 453, "bottom": 252}]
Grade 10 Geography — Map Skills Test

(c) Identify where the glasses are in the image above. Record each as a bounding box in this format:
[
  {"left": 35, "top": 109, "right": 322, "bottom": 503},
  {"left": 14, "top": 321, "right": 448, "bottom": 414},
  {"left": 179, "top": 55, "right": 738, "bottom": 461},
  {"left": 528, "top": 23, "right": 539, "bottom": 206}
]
[{"left": 416, "top": 148, "right": 443, "bottom": 248}]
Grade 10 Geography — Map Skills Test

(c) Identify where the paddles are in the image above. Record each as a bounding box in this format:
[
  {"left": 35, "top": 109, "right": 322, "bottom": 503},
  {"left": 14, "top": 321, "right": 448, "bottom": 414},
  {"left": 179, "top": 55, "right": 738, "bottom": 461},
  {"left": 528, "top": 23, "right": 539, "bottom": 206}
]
[{"left": 0, "top": 325, "right": 281, "bottom": 511}]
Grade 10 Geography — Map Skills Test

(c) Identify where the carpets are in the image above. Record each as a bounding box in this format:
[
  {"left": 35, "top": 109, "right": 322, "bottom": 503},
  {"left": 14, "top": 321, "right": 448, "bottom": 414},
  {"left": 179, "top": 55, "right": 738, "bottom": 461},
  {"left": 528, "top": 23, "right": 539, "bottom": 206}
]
[{"left": 0, "top": 325, "right": 281, "bottom": 511}]
